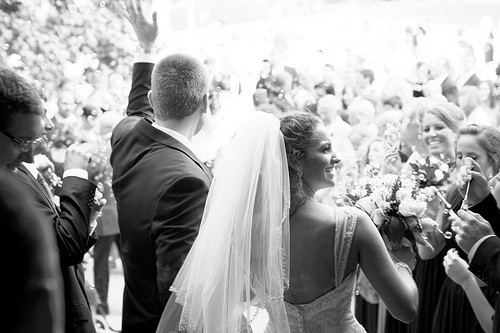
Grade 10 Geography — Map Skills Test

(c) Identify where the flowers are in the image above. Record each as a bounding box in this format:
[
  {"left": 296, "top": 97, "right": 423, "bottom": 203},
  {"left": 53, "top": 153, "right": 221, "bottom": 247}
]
[{"left": 334, "top": 163, "right": 452, "bottom": 305}]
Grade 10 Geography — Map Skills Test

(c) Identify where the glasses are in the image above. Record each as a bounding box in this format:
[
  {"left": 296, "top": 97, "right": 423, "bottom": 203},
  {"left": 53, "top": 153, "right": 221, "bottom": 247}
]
[{"left": 0, "top": 128, "right": 46, "bottom": 152}]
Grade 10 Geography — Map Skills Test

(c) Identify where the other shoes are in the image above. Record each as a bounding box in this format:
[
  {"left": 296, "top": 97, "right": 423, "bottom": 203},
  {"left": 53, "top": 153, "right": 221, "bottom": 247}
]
[{"left": 97, "top": 304, "right": 109, "bottom": 315}]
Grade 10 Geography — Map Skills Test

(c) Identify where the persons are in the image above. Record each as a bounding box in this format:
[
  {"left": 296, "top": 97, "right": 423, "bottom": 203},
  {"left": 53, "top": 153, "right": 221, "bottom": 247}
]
[{"left": 0, "top": 0, "right": 500, "bottom": 333}]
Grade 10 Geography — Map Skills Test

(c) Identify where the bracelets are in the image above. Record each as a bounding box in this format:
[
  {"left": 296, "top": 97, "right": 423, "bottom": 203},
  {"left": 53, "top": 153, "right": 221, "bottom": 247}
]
[
  {"left": 136, "top": 44, "right": 152, "bottom": 55},
  {"left": 396, "top": 263, "right": 412, "bottom": 276}
]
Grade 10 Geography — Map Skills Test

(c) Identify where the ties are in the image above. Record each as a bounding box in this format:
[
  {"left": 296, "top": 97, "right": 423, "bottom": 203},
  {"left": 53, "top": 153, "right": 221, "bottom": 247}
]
[{"left": 36, "top": 173, "right": 53, "bottom": 204}]
